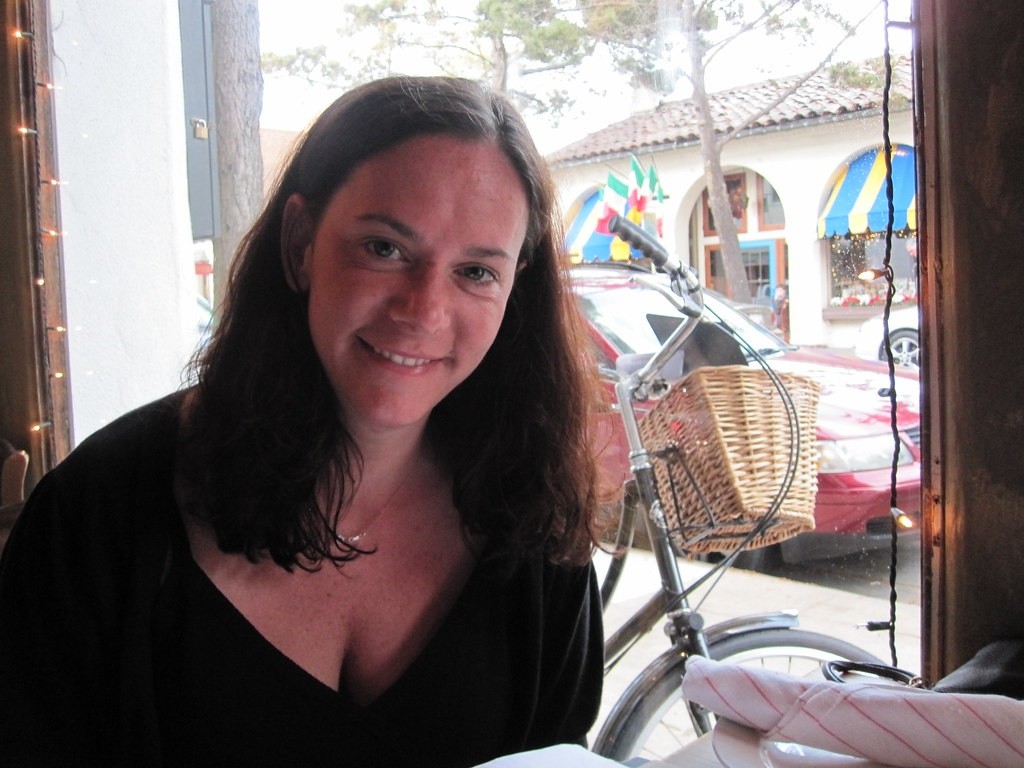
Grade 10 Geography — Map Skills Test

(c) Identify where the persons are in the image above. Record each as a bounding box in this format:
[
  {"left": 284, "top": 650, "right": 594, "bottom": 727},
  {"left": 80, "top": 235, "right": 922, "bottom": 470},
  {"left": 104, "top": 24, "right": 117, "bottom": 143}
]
[{"left": 1, "top": 73, "right": 619, "bottom": 768}]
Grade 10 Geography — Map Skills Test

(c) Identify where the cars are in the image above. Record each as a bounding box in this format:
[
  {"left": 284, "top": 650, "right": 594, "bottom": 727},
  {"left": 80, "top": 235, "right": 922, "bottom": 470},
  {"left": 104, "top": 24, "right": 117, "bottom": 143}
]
[{"left": 855, "top": 306, "right": 919, "bottom": 367}]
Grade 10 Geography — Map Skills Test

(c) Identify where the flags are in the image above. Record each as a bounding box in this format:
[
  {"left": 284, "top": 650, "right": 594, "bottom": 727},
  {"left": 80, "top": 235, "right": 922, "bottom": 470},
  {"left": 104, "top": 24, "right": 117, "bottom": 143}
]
[{"left": 602, "top": 155, "right": 664, "bottom": 202}]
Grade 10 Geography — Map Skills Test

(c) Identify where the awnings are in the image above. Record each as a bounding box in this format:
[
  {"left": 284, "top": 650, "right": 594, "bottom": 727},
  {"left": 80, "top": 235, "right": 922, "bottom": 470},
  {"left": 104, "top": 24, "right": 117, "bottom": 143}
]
[
  {"left": 814, "top": 143, "right": 921, "bottom": 242},
  {"left": 556, "top": 184, "right": 661, "bottom": 269}
]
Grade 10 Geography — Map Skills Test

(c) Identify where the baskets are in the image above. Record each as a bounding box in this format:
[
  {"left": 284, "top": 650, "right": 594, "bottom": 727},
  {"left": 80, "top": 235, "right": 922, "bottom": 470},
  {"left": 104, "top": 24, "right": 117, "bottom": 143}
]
[{"left": 634, "top": 364, "right": 820, "bottom": 555}]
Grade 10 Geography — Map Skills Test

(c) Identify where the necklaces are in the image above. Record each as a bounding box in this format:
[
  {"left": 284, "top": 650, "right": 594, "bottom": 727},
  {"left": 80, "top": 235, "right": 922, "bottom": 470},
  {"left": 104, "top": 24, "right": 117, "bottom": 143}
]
[{"left": 330, "top": 450, "right": 426, "bottom": 543}]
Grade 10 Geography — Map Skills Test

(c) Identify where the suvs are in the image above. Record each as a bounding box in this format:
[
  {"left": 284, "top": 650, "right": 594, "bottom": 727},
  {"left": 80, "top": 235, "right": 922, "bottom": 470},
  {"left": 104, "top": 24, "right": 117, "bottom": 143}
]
[{"left": 562, "top": 262, "right": 920, "bottom": 573}]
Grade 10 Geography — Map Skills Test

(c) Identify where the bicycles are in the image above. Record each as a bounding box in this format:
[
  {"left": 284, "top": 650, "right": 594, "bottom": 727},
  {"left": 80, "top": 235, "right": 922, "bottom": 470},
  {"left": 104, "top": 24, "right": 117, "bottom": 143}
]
[{"left": 588, "top": 215, "right": 909, "bottom": 766}]
